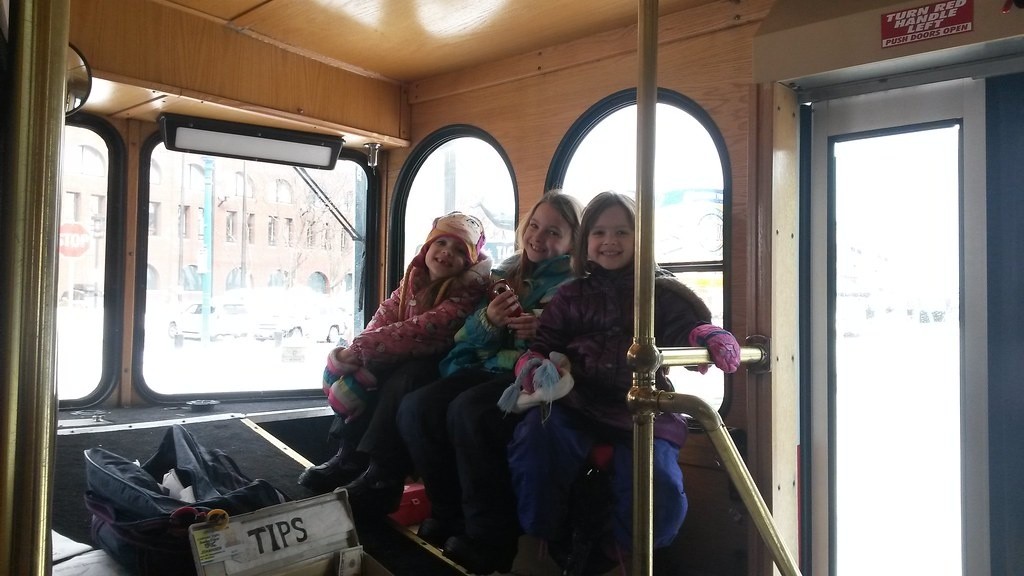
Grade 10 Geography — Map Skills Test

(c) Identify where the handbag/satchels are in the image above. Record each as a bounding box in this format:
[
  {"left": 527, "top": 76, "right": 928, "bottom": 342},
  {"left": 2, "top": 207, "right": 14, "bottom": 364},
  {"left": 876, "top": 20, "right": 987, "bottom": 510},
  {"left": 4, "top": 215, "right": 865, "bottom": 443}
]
[{"left": 82, "top": 424, "right": 287, "bottom": 576}]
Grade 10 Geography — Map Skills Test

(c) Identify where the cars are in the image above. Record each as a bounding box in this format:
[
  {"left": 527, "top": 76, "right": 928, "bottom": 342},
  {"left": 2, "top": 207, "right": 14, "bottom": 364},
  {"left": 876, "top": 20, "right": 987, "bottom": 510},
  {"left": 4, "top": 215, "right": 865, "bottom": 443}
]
[
  {"left": 254, "top": 307, "right": 347, "bottom": 343},
  {"left": 168, "top": 300, "right": 250, "bottom": 342}
]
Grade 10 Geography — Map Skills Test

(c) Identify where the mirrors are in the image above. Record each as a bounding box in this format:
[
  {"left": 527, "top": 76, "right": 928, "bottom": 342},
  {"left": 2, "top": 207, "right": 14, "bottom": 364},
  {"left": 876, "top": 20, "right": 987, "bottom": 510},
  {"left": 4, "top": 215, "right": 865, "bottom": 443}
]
[{"left": 65, "top": 42, "right": 93, "bottom": 118}]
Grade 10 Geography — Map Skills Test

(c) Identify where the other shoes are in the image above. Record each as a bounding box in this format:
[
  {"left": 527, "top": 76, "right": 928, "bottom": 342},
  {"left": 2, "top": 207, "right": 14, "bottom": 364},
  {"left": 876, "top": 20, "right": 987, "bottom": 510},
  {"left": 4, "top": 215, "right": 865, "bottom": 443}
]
[
  {"left": 441, "top": 535, "right": 509, "bottom": 574},
  {"left": 514, "top": 534, "right": 561, "bottom": 576},
  {"left": 605, "top": 555, "right": 629, "bottom": 576},
  {"left": 297, "top": 440, "right": 358, "bottom": 491},
  {"left": 419, "top": 516, "right": 464, "bottom": 545},
  {"left": 335, "top": 458, "right": 404, "bottom": 510}
]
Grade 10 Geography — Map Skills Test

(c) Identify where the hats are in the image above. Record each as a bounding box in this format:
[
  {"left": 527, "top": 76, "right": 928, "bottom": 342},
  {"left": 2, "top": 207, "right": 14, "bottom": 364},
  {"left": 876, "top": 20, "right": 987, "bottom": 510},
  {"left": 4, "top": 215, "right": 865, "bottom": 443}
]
[
  {"left": 497, "top": 351, "right": 574, "bottom": 422},
  {"left": 397, "top": 211, "right": 484, "bottom": 321}
]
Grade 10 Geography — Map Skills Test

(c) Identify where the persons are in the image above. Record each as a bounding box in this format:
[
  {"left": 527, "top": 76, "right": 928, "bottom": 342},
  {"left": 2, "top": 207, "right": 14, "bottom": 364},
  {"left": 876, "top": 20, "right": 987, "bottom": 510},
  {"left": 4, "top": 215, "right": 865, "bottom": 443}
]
[
  {"left": 505, "top": 192, "right": 740, "bottom": 576},
  {"left": 394, "top": 189, "right": 586, "bottom": 563},
  {"left": 297, "top": 210, "right": 493, "bottom": 502}
]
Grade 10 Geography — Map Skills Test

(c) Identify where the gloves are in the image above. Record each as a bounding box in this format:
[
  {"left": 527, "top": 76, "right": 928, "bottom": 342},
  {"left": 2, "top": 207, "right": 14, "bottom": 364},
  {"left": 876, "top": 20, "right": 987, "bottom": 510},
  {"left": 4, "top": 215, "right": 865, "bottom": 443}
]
[
  {"left": 323, "top": 347, "right": 359, "bottom": 396},
  {"left": 328, "top": 366, "right": 377, "bottom": 423},
  {"left": 515, "top": 351, "right": 546, "bottom": 391},
  {"left": 690, "top": 323, "right": 740, "bottom": 374}
]
